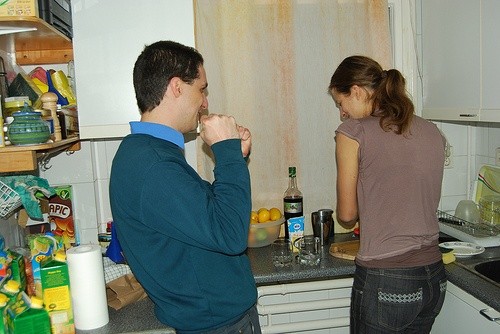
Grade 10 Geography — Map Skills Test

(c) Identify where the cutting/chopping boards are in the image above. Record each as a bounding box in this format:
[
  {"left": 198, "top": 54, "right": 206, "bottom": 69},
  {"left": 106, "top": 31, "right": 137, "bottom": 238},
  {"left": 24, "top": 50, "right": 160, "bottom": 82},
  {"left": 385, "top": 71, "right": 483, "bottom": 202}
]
[{"left": 329, "top": 240, "right": 360, "bottom": 261}]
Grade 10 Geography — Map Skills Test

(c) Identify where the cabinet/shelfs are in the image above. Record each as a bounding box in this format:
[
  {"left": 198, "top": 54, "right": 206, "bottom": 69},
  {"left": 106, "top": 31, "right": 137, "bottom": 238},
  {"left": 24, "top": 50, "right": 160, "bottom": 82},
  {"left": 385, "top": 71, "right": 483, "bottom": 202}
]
[
  {"left": 0, "top": 15, "right": 79, "bottom": 172},
  {"left": 416, "top": 0, "right": 500, "bottom": 123}
]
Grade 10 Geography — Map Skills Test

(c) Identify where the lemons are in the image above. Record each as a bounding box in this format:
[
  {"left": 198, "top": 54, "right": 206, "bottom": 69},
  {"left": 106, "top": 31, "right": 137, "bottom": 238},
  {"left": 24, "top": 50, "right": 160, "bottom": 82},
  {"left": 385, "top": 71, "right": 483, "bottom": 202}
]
[{"left": 248, "top": 208, "right": 281, "bottom": 246}]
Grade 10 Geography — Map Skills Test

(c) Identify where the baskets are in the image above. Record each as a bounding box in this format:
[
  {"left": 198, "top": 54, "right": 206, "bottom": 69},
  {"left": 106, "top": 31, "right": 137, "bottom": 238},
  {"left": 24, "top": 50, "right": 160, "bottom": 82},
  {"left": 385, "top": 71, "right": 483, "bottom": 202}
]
[{"left": 0, "top": 181, "right": 37, "bottom": 219}]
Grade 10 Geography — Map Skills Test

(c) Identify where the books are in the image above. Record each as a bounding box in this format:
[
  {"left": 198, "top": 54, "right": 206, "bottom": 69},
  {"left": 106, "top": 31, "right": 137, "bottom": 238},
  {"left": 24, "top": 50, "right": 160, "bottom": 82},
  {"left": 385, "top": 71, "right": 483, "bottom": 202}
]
[{"left": 8, "top": 72, "right": 42, "bottom": 105}]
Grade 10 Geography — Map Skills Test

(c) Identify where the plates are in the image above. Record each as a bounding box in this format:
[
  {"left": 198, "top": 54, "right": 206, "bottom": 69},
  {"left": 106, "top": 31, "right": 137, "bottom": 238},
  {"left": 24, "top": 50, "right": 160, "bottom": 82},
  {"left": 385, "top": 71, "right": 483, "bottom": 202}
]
[{"left": 438, "top": 241, "right": 485, "bottom": 258}]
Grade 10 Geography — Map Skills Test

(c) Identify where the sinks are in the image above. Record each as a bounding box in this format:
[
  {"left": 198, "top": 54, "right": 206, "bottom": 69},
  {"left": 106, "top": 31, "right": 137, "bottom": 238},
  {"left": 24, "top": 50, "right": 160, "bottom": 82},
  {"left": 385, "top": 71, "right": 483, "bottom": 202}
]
[{"left": 473, "top": 260, "right": 500, "bottom": 285}]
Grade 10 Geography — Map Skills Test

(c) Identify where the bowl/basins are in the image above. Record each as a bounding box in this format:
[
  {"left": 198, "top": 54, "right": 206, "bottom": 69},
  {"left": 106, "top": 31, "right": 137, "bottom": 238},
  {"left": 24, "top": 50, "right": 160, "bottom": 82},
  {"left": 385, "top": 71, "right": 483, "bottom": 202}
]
[
  {"left": 247, "top": 211, "right": 286, "bottom": 248},
  {"left": 454, "top": 200, "right": 480, "bottom": 225}
]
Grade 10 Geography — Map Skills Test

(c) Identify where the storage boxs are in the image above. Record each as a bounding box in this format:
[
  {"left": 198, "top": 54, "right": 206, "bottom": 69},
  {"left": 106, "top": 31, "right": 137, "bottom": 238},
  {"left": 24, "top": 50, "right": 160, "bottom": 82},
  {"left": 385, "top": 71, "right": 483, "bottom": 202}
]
[
  {"left": 0, "top": 0, "right": 40, "bottom": 18},
  {"left": 38, "top": 0, "right": 73, "bottom": 39},
  {"left": 47, "top": 185, "right": 80, "bottom": 247}
]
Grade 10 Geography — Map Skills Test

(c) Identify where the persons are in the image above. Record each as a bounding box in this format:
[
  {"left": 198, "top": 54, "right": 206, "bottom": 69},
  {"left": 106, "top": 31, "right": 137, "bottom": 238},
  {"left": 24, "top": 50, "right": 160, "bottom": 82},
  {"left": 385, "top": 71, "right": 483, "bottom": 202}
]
[
  {"left": 109, "top": 41, "right": 261, "bottom": 334},
  {"left": 329, "top": 56, "right": 447, "bottom": 334}
]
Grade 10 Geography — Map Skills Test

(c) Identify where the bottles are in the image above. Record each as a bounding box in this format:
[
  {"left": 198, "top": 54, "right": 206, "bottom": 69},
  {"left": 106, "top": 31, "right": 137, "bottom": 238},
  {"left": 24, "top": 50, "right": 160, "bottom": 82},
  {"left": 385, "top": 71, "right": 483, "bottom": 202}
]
[
  {"left": 282, "top": 166, "right": 303, "bottom": 240},
  {"left": 311, "top": 209, "right": 334, "bottom": 244},
  {"left": 3, "top": 100, "right": 50, "bottom": 146},
  {"left": 55, "top": 104, "right": 67, "bottom": 139}
]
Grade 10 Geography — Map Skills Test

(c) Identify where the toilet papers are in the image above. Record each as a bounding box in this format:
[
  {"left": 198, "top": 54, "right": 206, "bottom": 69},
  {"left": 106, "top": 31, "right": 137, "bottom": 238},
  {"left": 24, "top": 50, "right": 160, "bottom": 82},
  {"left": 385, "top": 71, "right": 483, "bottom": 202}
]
[{"left": 66, "top": 244, "right": 110, "bottom": 330}]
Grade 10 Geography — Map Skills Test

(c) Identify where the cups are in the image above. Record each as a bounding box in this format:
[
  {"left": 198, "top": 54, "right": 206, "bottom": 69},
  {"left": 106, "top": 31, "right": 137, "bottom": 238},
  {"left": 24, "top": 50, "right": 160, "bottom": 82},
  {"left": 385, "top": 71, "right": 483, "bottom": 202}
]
[
  {"left": 297, "top": 236, "right": 321, "bottom": 267},
  {"left": 270, "top": 239, "right": 293, "bottom": 268}
]
